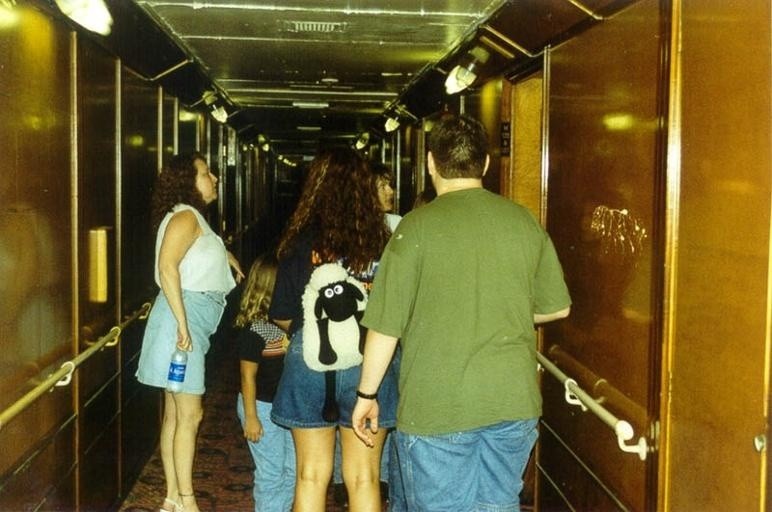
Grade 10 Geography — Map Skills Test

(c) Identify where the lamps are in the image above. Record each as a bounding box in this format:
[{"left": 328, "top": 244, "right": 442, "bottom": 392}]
[
  {"left": 200, "top": 88, "right": 230, "bottom": 125},
  {"left": 440, "top": 45, "right": 490, "bottom": 98},
  {"left": 383, "top": 105, "right": 409, "bottom": 131}
]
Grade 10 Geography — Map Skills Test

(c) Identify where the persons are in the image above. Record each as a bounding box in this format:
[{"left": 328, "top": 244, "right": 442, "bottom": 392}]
[
  {"left": 411, "top": 189, "right": 436, "bottom": 210},
  {"left": 233, "top": 250, "right": 296, "bottom": 511},
  {"left": 366, "top": 159, "right": 404, "bottom": 234},
  {"left": 132, "top": 149, "right": 247, "bottom": 512},
  {"left": 351, "top": 113, "right": 573, "bottom": 511},
  {"left": 269, "top": 147, "right": 396, "bottom": 512}
]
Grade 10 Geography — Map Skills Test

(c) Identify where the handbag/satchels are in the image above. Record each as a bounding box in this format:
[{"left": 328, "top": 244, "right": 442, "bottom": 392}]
[{"left": 302, "top": 262, "right": 369, "bottom": 372}]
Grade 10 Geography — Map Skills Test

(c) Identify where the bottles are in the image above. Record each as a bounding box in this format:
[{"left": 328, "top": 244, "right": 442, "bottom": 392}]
[{"left": 165, "top": 348, "right": 188, "bottom": 395}]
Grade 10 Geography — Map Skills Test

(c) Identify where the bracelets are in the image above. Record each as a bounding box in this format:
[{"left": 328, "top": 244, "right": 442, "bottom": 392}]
[{"left": 356, "top": 389, "right": 378, "bottom": 401}]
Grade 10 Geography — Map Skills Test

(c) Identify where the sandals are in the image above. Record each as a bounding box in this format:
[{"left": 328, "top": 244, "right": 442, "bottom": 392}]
[{"left": 160, "top": 497, "right": 182, "bottom": 512}]
